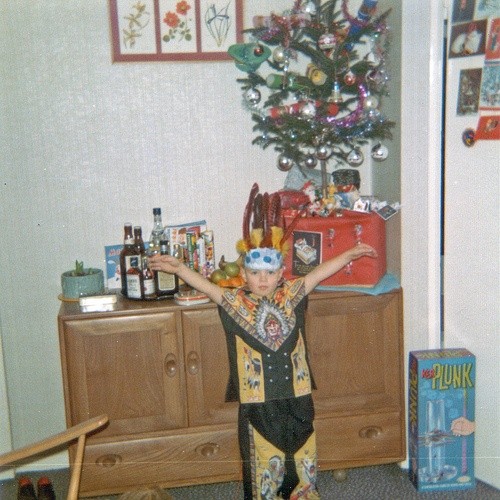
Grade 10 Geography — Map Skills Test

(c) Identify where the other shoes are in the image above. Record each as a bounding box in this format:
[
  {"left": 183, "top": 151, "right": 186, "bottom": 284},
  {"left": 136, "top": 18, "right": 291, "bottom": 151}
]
[
  {"left": 18, "top": 478, "right": 37, "bottom": 500},
  {"left": 37, "top": 478, "right": 56, "bottom": 500}
]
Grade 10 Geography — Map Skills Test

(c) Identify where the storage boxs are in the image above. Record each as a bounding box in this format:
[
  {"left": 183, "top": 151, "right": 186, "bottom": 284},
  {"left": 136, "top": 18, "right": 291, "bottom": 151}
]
[
  {"left": 408, "top": 349, "right": 475, "bottom": 492},
  {"left": 279, "top": 211, "right": 386, "bottom": 289}
]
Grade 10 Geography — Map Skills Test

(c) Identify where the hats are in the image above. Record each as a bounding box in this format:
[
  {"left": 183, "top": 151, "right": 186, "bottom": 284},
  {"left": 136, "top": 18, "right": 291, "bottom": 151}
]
[{"left": 240, "top": 181, "right": 311, "bottom": 269}]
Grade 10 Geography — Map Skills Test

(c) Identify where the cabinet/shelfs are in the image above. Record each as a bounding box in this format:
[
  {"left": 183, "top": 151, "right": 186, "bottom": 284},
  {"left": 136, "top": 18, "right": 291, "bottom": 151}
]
[{"left": 58, "top": 275, "right": 406, "bottom": 496}]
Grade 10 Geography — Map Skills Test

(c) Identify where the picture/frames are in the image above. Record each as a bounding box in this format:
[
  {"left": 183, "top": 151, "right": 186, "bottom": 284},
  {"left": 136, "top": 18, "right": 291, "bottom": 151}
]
[{"left": 109, "top": 0, "right": 244, "bottom": 62}]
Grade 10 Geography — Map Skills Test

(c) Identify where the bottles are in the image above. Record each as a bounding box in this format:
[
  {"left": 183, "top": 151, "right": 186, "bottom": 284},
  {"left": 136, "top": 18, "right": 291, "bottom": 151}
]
[{"left": 120, "top": 208, "right": 180, "bottom": 298}]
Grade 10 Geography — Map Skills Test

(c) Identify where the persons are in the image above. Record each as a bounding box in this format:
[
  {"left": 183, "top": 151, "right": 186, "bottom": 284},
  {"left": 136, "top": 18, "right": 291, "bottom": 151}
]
[{"left": 147, "top": 183, "right": 378, "bottom": 500}]
